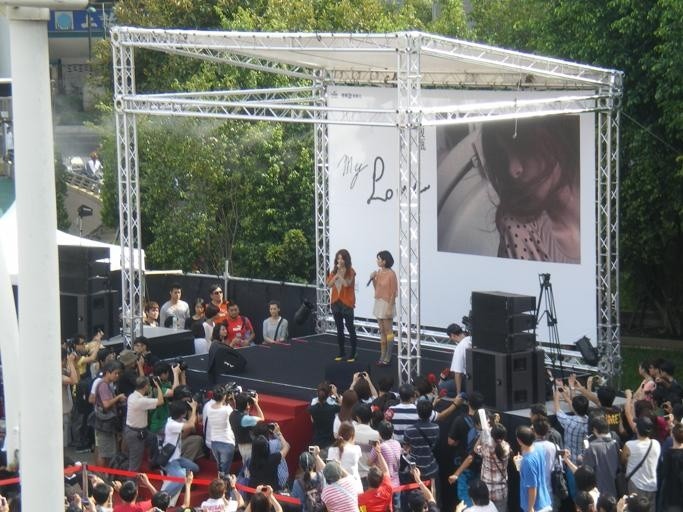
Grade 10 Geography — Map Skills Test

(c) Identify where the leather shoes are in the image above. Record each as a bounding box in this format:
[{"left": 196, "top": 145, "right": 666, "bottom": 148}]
[
  {"left": 376, "top": 358, "right": 391, "bottom": 366},
  {"left": 334, "top": 353, "right": 346, "bottom": 360},
  {"left": 347, "top": 353, "right": 359, "bottom": 362}
]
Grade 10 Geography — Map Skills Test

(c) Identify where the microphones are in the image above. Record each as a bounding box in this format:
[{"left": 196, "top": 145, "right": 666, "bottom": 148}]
[
  {"left": 333, "top": 263, "right": 341, "bottom": 274},
  {"left": 367, "top": 271, "right": 377, "bottom": 286}
]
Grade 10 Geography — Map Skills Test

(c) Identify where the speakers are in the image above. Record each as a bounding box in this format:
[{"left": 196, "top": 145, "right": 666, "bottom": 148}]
[{"left": 466, "top": 292, "right": 545, "bottom": 411}]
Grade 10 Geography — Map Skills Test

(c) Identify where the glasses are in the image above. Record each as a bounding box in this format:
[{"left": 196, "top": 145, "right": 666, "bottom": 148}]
[{"left": 214, "top": 290, "right": 224, "bottom": 295}]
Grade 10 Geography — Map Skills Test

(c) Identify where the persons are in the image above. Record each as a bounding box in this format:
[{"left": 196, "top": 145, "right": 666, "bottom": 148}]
[
  {"left": 369, "top": 249, "right": 398, "bottom": 367},
  {"left": 325, "top": 248, "right": 358, "bottom": 364},
  {"left": 478, "top": 115, "right": 580, "bottom": 265},
  {"left": 1, "top": 282, "right": 683, "bottom": 512},
  {"left": 85, "top": 150, "right": 103, "bottom": 181}
]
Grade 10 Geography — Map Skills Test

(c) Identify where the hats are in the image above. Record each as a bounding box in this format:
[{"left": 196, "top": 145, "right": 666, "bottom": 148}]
[{"left": 633, "top": 417, "right": 652, "bottom": 431}]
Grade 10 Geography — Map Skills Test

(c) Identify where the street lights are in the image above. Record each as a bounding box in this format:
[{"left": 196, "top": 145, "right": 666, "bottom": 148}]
[{"left": 81, "top": 5, "right": 100, "bottom": 96}]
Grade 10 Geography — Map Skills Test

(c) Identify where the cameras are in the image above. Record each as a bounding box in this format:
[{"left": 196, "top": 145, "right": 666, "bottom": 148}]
[
  {"left": 557, "top": 451, "right": 565, "bottom": 455},
  {"left": 663, "top": 415, "right": 669, "bottom": 421},
  {"left": 558, "top": 387, "right": 564, "bottom": 392},
  {"left": 592, "top": 377, "right": 598, "bottom": 381},
  {"left": 61, "top": 328, "right": 381, "bottom": 493}
]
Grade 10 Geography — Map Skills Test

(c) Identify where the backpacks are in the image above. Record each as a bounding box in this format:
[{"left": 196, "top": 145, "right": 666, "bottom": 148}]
[
  {"left": 75, "top": 376, "right": 100, "bottom": 413},
  {"left": 298, "top": 471, "right": 326, "bottom": 511},
  {"left": 465, "top": 416, "right": 480, "bottom": 451}
]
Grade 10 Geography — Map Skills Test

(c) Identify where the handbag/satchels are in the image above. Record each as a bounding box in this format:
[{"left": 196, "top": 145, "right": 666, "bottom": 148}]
[
  {"left": 552, "top": 470, "right": 569, "bottom": 500},
  {"left": 95, "top": 402, "right": 115, "bottom": 419},
  {"left": 615, "top": 473, "right": 628, "bottom": 497},
  {"left": 155, "top": 444, "right": 177, "bottom": 466}
]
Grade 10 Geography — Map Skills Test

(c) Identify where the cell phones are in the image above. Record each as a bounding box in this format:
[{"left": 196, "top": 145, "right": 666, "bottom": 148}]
[
  {"left": 583, "top": 437, "right": 589, "bottom": 450},
  {"left": 411, "top": 462, "right": 416, "bottom": 470}
]
